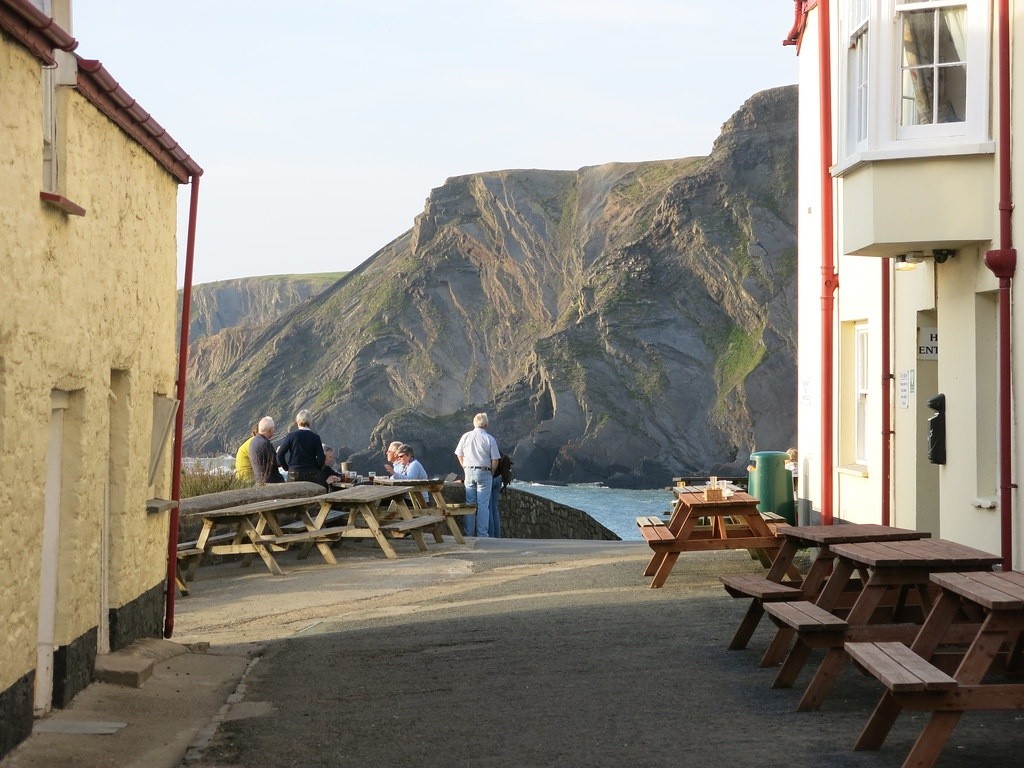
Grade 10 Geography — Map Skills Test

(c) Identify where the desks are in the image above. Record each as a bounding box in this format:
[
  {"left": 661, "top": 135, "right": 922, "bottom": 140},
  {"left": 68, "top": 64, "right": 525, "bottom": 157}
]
[
  {"left": 177, "top": 477, "right": 466, "bottom": 597},
  {"left": 635, "top": 474, "right": 1024, "bottom": 767}
]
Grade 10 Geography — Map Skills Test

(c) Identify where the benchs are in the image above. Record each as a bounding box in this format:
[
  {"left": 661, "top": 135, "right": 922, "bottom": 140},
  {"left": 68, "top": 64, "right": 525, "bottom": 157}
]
[
  {"left": 764, "top": 523, "right": 793, "bottom": 548},
  {"left": 280, "top": 509, "right": 350, "bottom": 531},
  {"left": 718, "top": 575, "right": 804, "bottom": 608},
  {"left": 276, "top": 525, "right": 355, "bottom": 552},
  {"left": 446, "top": 503, "right": 477, "bottom": 516},
  {"left": 377, "top": 515, "right": 447, "bottom": 534},
  {"left": 638, "top": 527, "right": 677, "bottom": 554},
  {"left": 761, "top": 599, "right": 850, "bottom": 647},
  {"left": 842, "top": 641, "right": 959, "bottom": 713},
  {"left": 177, "top": 531, "right": 242, "bottom": 553},
  {"left": 636, "top": 516, "right": 665, "bottom": 528},
  {"left": 760, "top": 511, "right": 786, "bottom": 524},
  {"left": 175, "top": 549, "right": 205, "bottom": 599}
]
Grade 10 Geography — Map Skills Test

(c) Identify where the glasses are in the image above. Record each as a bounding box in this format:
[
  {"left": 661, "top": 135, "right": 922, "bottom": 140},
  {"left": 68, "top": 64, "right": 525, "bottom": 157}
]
[{"left": 398, "top": 454, "right": 406, "bottom": 458}]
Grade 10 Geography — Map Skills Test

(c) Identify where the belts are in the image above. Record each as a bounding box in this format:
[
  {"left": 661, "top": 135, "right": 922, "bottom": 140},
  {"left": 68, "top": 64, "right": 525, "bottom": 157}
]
[{"left": 471, "top": 466, "right": 490, "bottom": 470}]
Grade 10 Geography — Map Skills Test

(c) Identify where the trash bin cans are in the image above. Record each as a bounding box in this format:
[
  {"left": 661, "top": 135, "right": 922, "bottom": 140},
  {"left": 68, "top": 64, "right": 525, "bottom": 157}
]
[{"left": 747, "top": 450, "right": 796, "bottom": 527}]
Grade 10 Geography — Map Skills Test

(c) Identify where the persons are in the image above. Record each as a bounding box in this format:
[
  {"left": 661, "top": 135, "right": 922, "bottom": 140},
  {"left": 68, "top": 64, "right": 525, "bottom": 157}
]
[
  {"left": 454, "top": 411, "right": 503, "bottom": 538},
  {"left": 235, "top": 416, "right": 431, "bottom": 508},
  {"left": 785, "top": 447, "right": 798, "bottom": 501},
  {"left": 278, "top": 410, "right": 326, "bottom": 486}
]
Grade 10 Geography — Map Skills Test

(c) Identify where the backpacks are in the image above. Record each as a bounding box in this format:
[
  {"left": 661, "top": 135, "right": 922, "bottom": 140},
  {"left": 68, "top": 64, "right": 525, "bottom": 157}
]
[{"left": 499, "top": 451, "right": 514, "bottom": 495}]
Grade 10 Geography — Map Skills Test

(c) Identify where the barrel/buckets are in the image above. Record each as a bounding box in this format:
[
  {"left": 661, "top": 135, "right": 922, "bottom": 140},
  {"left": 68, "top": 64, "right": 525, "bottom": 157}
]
[{"left": 340, "top": 461, "right": 353, "bottom": 474}]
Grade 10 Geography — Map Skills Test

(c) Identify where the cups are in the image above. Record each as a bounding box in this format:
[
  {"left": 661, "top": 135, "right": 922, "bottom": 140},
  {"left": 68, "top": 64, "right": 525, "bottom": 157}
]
[
  {"left": 357, "top": 475, "right": 363, "bottom": 483},
  {"left": 709, "top": 476, "right": 717, "bottom": 489},
  {"left": 386, "top": 464, "right": 391, "bottom": 471},
  {"left": 719, "top": 480, "right": 727, "bottom": 500},
  {"left": 350, "top": 472, "right": 356, "bottom": 483},
  {"left": 369, "top": 472, "right": 375, "bottom": 482}
]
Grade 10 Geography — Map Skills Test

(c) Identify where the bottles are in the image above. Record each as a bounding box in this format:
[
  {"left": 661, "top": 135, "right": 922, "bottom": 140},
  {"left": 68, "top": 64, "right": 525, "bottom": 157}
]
[{"left": 342, "top": 470, "right": 350, "bottom": 484}]
